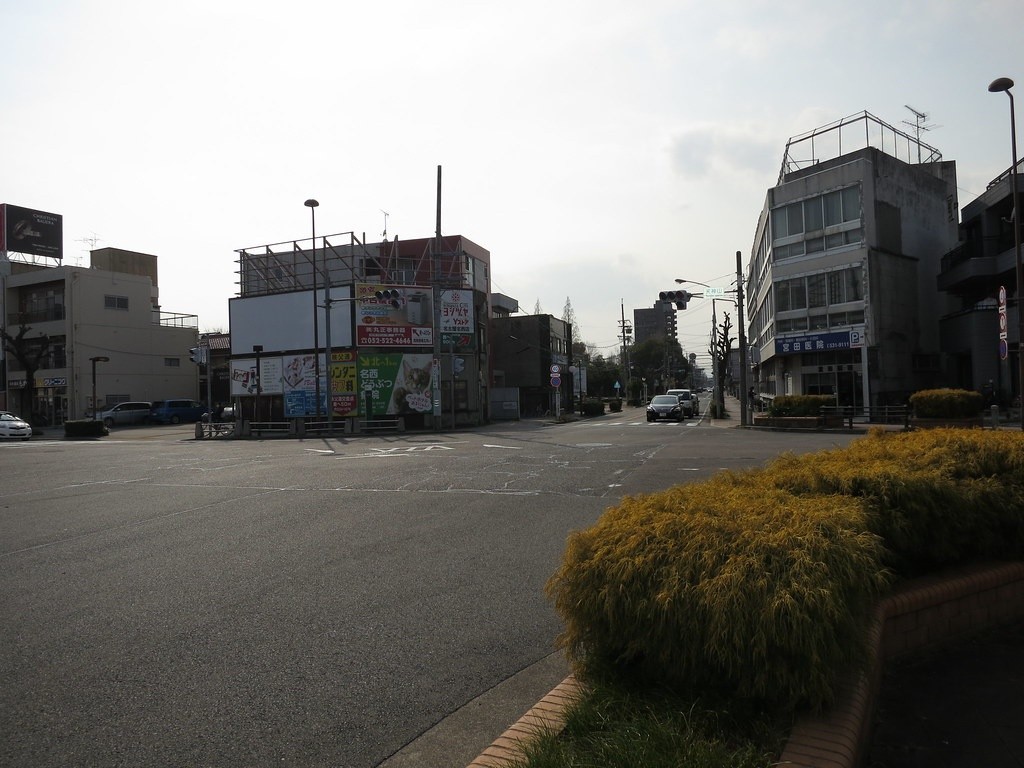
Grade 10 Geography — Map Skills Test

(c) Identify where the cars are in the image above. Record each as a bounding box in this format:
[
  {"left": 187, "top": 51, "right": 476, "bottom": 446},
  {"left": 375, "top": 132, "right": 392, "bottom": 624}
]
[
  {"left": 691, "top": 394, "right": 701, "bottom": 417},
  {"left": 151, "top": 399, "right": 207, "bottom": 424},
  {"left": 646, "top": 395, "right": 685, "bottom": 422},
  {"left": 0, "top": 411, "right": 33, "bottom": 441},
  {"left": 696, "top": 387, "right": 713, "bottom": 393},
  {"left": 96, "top": 401, "right": 153, "bottom": 428}
]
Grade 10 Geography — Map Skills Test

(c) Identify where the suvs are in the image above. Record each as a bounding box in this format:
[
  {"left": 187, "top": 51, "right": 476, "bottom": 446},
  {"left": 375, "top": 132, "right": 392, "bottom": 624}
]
[{"left": 666, "top": 388, "right": 696, "bottom": 419}]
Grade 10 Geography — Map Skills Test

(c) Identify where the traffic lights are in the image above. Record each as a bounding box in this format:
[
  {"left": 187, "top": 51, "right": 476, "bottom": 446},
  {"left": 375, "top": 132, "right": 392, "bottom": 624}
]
[
  {"left": 189, "top": 346, "right": 206, "bottom": 364},
  {"left": 452, "top": 357, "right": 465, "bottom": 377},
  {"left": 660, "top": 289, "right": 691, "bottom": 311},
  {"left": 374, "top": 289, "right": 403, "bottom": 310}
]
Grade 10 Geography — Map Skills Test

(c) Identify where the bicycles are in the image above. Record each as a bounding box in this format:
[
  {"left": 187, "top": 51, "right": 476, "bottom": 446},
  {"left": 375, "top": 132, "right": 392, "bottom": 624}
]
[{"left": 201, "top": 409, "right": 235, "bottom": 437}]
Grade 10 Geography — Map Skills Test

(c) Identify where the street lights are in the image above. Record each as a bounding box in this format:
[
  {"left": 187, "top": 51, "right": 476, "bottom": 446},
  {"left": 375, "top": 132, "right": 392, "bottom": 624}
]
[
  {"left": 90, "top": 355, "right": 110, "bottom": 419},
  {"left": 673, "top": 278, "right": 721, "bottom": 419},
  {"left": 304, "top": 198, "right": 319, "bottom": 434},
  {"left": 985, "top": 76, "right": 1024, "bottom": 431}
]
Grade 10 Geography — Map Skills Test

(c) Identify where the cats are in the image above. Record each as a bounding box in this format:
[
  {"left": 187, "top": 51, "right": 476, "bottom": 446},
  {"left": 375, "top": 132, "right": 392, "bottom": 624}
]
[{"left": 392, "top": 359, "right": 432, "bottom": 412}]
